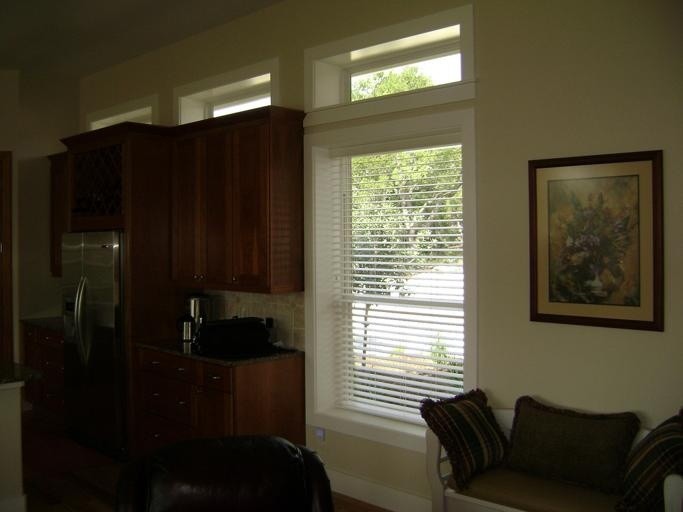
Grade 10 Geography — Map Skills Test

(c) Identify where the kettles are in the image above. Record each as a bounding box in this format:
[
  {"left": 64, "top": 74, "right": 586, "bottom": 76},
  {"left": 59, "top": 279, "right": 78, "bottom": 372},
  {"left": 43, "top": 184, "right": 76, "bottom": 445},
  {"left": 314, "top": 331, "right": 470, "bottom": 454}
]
[
  {"left": 176, "top": 314, "right": 196, "bottom": 343},
  {"left": 186, "top": 296, "right": 212, "bottom": 335}
]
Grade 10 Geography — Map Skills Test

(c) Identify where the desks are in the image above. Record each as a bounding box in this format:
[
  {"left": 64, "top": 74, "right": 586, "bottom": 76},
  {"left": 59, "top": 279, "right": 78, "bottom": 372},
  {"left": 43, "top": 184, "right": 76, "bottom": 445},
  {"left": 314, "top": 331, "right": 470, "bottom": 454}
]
[{"left": 0, "top": 360, "right": 43, "bottom": 511}]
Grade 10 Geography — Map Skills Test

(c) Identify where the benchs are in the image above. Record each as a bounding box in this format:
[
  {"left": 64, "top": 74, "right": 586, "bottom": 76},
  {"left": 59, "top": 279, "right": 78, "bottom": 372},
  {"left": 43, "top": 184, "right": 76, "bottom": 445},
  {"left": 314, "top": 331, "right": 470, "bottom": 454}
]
[{"left": 423, "top": 407, "right": 683, "bottom": 512}]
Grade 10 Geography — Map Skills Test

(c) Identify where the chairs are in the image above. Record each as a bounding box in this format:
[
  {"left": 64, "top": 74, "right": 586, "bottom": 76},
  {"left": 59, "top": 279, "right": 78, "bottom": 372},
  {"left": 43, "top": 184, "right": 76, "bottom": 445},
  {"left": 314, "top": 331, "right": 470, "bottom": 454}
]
[{"left": 111, "top": 432, "right": 338, "bottom": 512}]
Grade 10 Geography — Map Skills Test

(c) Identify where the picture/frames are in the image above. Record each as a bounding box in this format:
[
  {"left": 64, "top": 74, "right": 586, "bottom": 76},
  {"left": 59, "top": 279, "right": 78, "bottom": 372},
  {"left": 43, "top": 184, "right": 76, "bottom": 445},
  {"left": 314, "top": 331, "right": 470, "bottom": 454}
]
[{"left": 528, "top": 150, "right": 666, "bottom": 330}]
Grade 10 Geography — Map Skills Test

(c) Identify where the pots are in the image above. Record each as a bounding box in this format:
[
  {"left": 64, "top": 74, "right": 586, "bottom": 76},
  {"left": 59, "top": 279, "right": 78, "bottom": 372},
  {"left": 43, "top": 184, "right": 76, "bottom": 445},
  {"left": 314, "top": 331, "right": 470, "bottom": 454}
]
[{"left": 195, "top": 315, "right": 271, "bottom": 359}]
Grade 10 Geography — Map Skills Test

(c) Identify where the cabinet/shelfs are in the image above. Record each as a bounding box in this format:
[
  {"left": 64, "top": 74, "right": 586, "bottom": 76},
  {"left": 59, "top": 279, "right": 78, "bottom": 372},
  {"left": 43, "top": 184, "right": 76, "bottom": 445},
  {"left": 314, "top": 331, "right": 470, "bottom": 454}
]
[
  {"left": 21, "top": 317, "right": 69, "bottom": 435},
  {"left": 168, "top": 116, "right": 233, "bottom": 290},
  {"left": 232, "top": 108, "right": 308, "bottom": 293},
  {"left": 133, "top": 339, "right": 306, "bottom": 455},
  {"left": 60, "top": 137, "right": 168, "bottom": 228},
  {"left": 47, "top": 156, "right": 66, "bottom": 278}
]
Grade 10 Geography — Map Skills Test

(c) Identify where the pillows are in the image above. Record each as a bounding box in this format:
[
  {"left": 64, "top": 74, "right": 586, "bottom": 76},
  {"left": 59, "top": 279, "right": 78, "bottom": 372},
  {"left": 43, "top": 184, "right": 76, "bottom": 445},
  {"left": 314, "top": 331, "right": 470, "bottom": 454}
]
[
  {"left": 617, "top": 409, "right": 683, "bottom": 512},
  {"left": 508, "top": 395, "right": 642, "bottom": 488},
  {"left": 421, "top": 388, "right": 509, "bottom": 486}
]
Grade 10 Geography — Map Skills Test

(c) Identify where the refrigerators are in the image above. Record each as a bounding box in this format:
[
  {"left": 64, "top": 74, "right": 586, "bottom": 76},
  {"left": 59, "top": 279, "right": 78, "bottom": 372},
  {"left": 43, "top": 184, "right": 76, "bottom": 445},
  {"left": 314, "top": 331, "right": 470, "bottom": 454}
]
[{"left": 58, "top": 228, "right": 131, "bottom": 461}]
